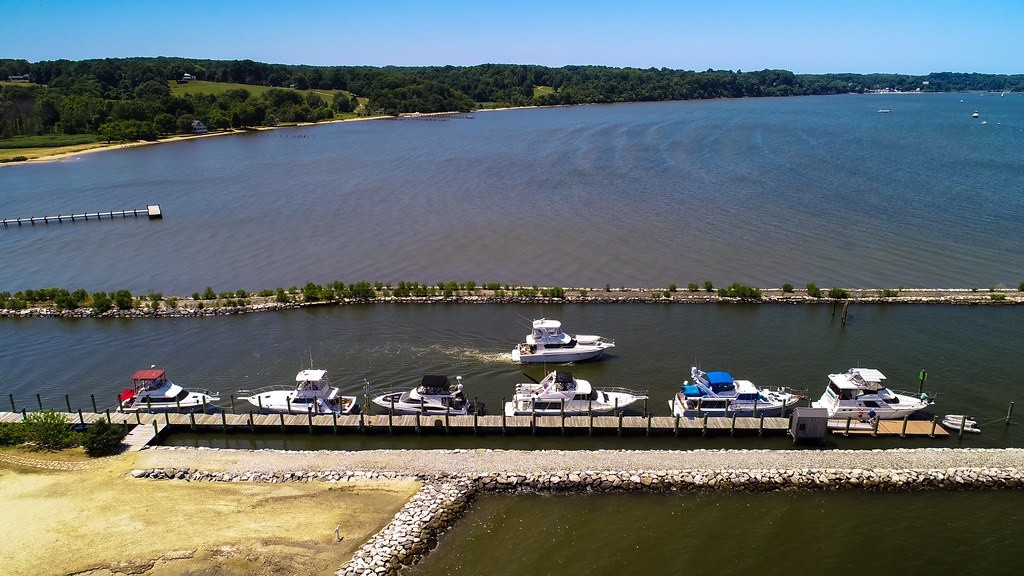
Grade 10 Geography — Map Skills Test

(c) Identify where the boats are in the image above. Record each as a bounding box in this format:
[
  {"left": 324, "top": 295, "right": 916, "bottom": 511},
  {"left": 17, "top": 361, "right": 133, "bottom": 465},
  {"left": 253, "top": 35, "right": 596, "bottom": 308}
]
[
  {"left": 511, "top": 311, "right": 616, "bottom": 363},
  {"left": 943, "top": 420, "right": 981, "bottom": 433},
  {"left": 235, "top": 347, "right": 361, "bottom": 415},
  {"left": 811, "top": 367, "right": 935, "bottom": 420},
  {"left": 668, "top": 359, "right": 808, "bottom": 418},
  {"left": 944, "top": 415, "right": 977, "bottom": 424},
  {"left": 116, "top": 364, "right": 221, "bottom": 415},
  {"left": 504, "top": 369, "right": 650, "bottom": 417},
  {"left": 369, "top": 374, "right": 471, "bottom": 416}
]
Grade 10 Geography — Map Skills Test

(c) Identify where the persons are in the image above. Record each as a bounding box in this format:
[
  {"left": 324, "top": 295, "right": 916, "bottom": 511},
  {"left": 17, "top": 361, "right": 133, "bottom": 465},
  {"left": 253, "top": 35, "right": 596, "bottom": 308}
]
[
  {"left": 316, "top": 400, "right": 323, "bottom": 412},
  {"left": 308, "top": 402, "right": 314, "bottom": 414}
]
